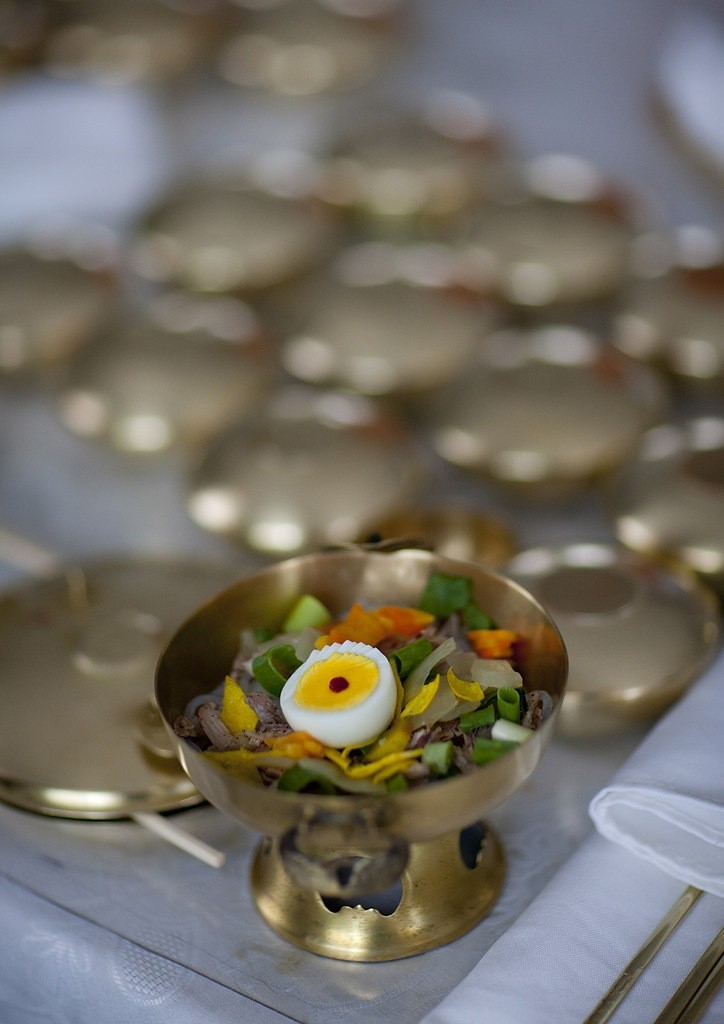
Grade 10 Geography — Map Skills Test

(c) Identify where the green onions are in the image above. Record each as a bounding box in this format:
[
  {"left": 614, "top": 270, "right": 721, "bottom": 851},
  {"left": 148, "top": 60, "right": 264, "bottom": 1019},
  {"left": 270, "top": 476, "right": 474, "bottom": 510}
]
[{"left": 185, "top": 567, "right": 535, "bottom": 794}]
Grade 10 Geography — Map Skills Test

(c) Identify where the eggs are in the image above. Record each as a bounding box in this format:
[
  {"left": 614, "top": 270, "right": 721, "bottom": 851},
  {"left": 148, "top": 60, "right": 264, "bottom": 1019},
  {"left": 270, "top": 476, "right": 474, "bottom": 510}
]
[{"left": 280, "top": 640, "right": 397, "bottom": 747}]
